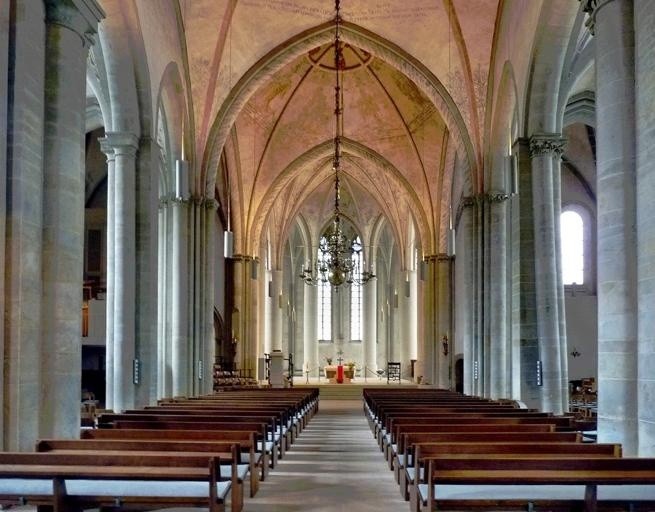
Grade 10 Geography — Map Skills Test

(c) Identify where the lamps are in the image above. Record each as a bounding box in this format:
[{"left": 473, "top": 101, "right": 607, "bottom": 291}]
[{"left": 300, "top": 2, "right": 378, "bottom": 287}]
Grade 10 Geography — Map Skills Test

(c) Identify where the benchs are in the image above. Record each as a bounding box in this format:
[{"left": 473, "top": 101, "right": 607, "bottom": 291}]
[{"left": 364, "top": 389, "right": 655, "bottom": 512}]
